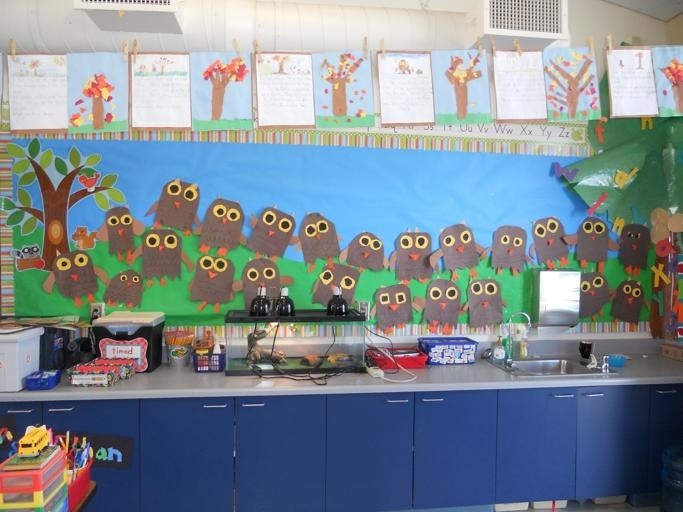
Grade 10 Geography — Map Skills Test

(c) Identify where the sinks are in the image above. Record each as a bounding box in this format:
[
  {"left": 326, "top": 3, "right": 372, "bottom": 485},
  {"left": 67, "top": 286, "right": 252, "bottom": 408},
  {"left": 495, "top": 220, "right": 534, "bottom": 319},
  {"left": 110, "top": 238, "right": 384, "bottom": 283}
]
[{"left": 487, "top": 356, "right": 620, "bottom": 378}]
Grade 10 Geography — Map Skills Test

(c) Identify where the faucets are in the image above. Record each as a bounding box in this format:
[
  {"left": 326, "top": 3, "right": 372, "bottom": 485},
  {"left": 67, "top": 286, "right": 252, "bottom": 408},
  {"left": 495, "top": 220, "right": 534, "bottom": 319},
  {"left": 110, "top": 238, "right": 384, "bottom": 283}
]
[{"left": 507, "top": 312, "right": 532, "bottom": 361}]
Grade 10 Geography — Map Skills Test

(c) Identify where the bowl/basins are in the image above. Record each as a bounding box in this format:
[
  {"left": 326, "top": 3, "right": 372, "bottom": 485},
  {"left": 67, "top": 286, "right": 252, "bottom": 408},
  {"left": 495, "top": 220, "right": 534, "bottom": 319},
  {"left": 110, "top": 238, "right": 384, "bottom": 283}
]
[{"left": 607, "top": 355, "right": 626, "bottom": 368}]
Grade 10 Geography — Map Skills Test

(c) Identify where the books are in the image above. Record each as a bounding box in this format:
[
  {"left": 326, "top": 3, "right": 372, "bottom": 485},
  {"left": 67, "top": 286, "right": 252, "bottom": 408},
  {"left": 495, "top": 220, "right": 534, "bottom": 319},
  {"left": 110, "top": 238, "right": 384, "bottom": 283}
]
[
  {"left": 10, "top": 318, "right": 64, "bottom": 328},
  {"left": 2, "top": 445, "right": 61, "bottom": 472},
  {"left": 0, "top": 322, "right": 34, "bottom": 335}
]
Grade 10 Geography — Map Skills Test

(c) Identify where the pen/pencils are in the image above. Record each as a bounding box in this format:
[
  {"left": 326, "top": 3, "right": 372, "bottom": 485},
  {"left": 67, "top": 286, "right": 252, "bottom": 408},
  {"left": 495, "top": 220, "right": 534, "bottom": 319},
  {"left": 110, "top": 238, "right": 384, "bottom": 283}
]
[
  {"left": 58, "top": 429, "right": 91, "bottom": 470},
  {"left": 165, "top": 325, "right": 197, "bottom": 344}
]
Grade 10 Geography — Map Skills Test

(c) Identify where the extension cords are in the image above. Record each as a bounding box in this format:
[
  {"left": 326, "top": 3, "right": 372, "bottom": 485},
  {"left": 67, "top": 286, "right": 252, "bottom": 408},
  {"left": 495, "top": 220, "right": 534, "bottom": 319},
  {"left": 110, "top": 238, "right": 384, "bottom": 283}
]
[{"left": 361, "top": 360, "right": 384, "bottom": 378}]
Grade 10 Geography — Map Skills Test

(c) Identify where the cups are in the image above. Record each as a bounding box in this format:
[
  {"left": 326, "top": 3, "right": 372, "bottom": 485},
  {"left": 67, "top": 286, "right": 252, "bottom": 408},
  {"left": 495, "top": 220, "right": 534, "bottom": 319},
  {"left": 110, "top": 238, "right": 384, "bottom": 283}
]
[{"left": 578, "top": 340, "right": 593, "bottom": 359}]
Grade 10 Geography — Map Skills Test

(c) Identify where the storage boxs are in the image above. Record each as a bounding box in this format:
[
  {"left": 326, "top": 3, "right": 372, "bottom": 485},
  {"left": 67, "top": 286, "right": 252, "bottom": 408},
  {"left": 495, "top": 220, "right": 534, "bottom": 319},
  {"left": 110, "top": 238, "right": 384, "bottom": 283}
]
[{"left": 0, "top": 319, "right": 165, "bottom": 393}]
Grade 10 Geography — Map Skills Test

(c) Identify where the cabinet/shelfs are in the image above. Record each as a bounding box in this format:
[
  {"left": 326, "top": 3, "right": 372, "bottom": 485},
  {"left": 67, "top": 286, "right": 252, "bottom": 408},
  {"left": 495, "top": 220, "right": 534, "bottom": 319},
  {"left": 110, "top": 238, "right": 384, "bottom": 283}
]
[{"left": 0, "top": 384, "right": 682, "bottom": 512}]
